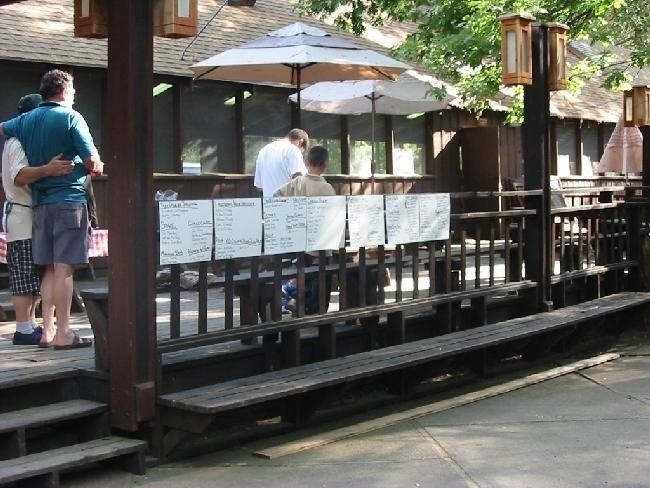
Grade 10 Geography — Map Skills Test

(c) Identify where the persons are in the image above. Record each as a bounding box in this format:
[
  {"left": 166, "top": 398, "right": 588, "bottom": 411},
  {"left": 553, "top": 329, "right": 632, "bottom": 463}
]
[
  {"left": 253, "top": 125, "right": 310, "bottom": 316},
  {"left": 0, "top": 68, "right": 105, "bottom": 350},
  {"left": 0, "top": 90, "right": 77, "bottom": 346},
  {"left": 270, "top": 145, "right": 339, "bottom": 316}
]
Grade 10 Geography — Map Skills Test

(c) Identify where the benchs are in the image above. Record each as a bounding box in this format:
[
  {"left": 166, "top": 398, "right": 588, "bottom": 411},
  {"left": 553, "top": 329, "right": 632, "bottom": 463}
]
[{"left": 79, "top": 229, "right": 649, "bottom": 454}]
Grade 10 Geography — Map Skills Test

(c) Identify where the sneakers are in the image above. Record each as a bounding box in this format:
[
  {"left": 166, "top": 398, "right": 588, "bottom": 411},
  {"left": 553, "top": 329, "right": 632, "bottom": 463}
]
[{"left": 281, "top": 283, "right": 296, "bottom": 314}]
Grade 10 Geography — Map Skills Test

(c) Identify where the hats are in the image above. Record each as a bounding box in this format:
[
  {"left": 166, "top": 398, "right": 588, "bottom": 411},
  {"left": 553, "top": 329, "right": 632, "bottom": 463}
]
[
  {"left": 17, "top": 93, "right": 42, "bottom": 113},
  {"left": 13, "top": 325, "right": 41, "bottom": 344}
]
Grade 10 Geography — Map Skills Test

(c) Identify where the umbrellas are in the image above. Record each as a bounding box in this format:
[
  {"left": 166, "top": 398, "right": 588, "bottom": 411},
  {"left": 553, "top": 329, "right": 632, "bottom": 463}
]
[
  {"left": 288, "top": 71, "right": 466, "bottom": 196},
  {"left": 184, "top": 20, "right": 410, "bottom": 129},
  {"left": 591, "top": 109, "right": 644, "bottom": 187}
]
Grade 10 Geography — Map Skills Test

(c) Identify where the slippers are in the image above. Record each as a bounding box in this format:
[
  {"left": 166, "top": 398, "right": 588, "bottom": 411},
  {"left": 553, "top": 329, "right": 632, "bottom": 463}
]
[
  {"left": 54, "top": 332, "right": 93, "bottom": 349},
  {"left": 40, "top": 335, "right": 57, "bottom": 347}
]
